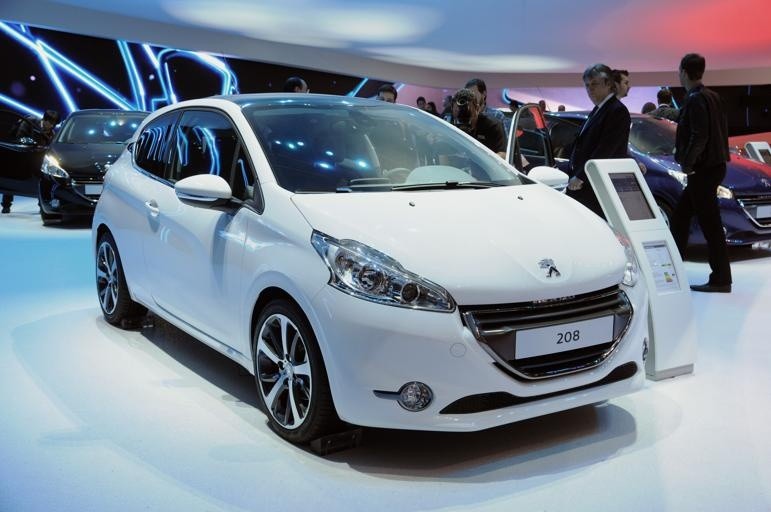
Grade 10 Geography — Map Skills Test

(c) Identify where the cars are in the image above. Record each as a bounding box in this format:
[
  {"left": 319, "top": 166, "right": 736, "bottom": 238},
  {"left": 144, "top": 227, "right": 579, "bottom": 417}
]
[
  {"left": 443, "top": 111, "right": 771, "bottom": 262},
  {"left": 1, "top": 108, "right": 154, "bottom": 227},
  {"left": 91, "top": 92, "right": 649, "bottom": 446}
]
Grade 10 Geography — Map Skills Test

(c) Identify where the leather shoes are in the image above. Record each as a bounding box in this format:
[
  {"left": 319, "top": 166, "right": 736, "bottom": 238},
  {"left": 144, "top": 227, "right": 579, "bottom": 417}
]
[{"left": 690, "top": 282, "right": 733, "bottom": 293}]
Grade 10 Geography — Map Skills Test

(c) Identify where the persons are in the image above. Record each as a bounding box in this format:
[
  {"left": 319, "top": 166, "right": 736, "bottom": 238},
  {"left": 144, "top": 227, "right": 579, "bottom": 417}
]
[
  {"left": 558, "top": 105, "right": 565, "bottom": 112},
  {"left": 0, "top": 110, "right": 61, "bottom": 213},
  {"left": 286, "top": 78, "right": 310, "bottom": 93},
  {"left": 566, "top": 64, "right": 647, "bottom": 219},
  {"left": 417, "top": 79, "right": 510, "bottom": 180},
  {"left": 509, "top": 100, "right": 518, "bottom": 112},
  {"left": 539, "top": 100, "right": 546, "bottom": 111},
  {"left": 378, "top": 83, "right": 398, "bottom": 102},
  {"left": 669, "top": 51, "right": 732, "bottom": 292},
  {"left": 643, "top": 88, "right": 679, "bottom": 124}
]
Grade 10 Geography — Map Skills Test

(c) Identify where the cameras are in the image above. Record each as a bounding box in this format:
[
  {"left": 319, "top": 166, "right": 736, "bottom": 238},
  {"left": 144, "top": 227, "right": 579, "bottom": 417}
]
[{"left": 453, "top": 96, "right": 472, "bottom": 126}]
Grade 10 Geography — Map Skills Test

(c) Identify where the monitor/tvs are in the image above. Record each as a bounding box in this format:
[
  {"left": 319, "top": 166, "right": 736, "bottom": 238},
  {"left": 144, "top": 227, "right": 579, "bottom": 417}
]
[
  {"left": 744, "top": 141, "right": 771, "bottom": 167},
  {"left": 585, "top": 158, "right": 668, "bottom": 233}
]
